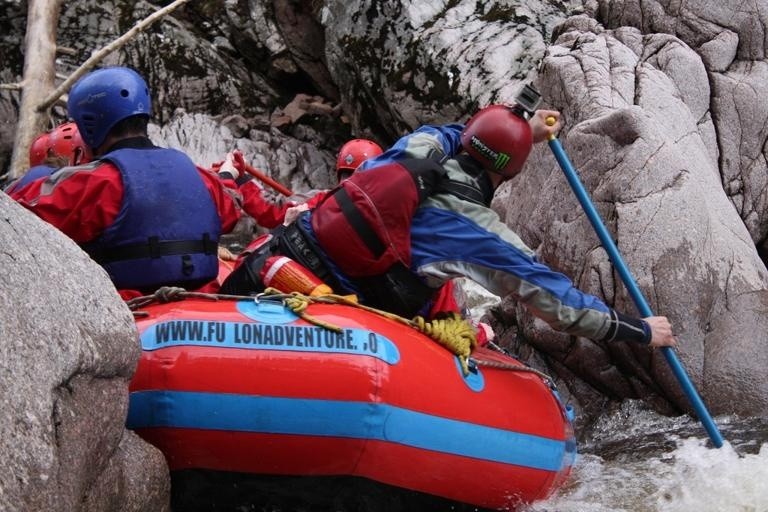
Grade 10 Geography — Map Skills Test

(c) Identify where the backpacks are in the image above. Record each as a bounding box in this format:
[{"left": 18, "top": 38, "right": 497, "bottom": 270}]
[{"left": 310, "top": 146, "right": 488, "bottom": 278}]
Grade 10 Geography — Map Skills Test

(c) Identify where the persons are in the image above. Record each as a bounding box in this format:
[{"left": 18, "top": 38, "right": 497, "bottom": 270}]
[
  {"left": 17, "top": 66, "right": 242, "bottom": 298},
  {"left": 4, "top": 123, "right": 90, "bottom": 204},
  {"left": 219, "top": 102, "right": 676, "bottom": 358},
  {"left": 231, "top": 138, "right": 382, "bottom": 226}
]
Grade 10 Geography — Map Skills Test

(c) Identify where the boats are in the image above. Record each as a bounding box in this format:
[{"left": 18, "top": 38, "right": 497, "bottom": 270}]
[{"left": 105, "top": 274, "right": 577, "bottom": 512}]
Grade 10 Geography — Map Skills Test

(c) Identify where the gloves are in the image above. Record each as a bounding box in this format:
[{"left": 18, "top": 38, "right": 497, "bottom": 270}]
[{"left": 218, "top": 149, "right": 240, "bottom": 180}]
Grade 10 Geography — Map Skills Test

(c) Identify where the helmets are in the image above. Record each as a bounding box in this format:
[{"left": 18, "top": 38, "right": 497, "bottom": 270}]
[
  {"left": 336, "top": 138, "right": 384, "bottom": 173},
  {"left": 68, "top": 66, "right": 153, "bottom": 148},
  {"left": 460, "top": 105, "right": 532, "bottom": 176},
  {"left": 28, "top": 122, "right": 93, "bottom": 168}
]
